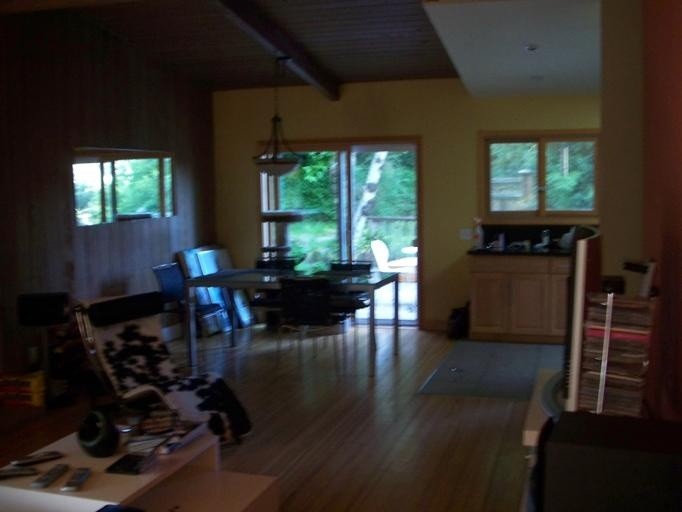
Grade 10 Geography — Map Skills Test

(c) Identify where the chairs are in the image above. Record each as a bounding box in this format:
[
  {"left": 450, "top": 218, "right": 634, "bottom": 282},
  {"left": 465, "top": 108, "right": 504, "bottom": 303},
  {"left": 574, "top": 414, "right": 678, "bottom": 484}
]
[
  {"left": 330, "top": 258, "right": 376, "bottom": 334},
  {"left": 75, "top": 287, "right": 244, "bottom": 448},
  {"left": 248, "top": 255, "right": 296, "bottom": 338},
  {"left": 153, "top": 260, "right": 225, "bottom": 356},
  {"left": 273, "top": 276, "right": 351, "bottom": 377}
]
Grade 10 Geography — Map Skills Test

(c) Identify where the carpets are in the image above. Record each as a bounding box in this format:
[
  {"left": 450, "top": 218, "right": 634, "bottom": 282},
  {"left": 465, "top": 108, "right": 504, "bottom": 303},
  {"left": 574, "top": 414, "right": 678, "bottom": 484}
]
[{"left": 414, "top": 336, "right": 569, "bottom": 403}]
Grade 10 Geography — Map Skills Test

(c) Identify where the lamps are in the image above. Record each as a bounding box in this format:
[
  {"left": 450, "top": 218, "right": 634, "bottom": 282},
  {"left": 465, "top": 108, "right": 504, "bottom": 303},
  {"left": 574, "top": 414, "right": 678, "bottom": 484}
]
[{"left": 241, "top": 53, "right": 304, "bottom": 183}]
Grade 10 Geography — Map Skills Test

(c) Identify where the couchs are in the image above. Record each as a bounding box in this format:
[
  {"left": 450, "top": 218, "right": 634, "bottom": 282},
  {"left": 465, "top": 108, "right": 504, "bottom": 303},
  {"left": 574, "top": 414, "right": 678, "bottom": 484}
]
[{"left": 527, "top": 408, "right": 680, "bottom": 511}]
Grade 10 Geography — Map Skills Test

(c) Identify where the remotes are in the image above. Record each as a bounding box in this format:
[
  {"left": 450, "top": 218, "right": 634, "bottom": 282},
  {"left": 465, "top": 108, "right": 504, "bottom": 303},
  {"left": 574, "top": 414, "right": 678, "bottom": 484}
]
[
  {"left": 9, "top": 451, "right": 63, "bottom": 467},
  {"left": 0, "top": 467, "right": 38, "bottom": 478},
  {"left": 32, "top": 464, "right": 67, "bottom": 487},
  {"left": 60, "top": 468, "right": 90, "bottom": 491}
]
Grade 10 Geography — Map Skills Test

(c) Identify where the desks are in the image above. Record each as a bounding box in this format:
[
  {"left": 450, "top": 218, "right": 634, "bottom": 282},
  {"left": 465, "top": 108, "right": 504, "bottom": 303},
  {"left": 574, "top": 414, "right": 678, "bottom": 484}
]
[
  {"left": 183, "top": 267, "right": 401, "bottom": 378},
  {"left": 524, "top": 364, "right": 571, "bottom": 466}
]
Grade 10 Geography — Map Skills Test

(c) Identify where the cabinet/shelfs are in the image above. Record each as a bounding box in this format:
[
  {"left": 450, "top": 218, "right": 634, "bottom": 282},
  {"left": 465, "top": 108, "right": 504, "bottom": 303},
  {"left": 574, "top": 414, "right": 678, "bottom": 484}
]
[
  {"left": 468, "top": 247, "right": 576, "bottom": 343},
  {"left": 580, "top": 292, "right": 656, "bottom": 417}
]
